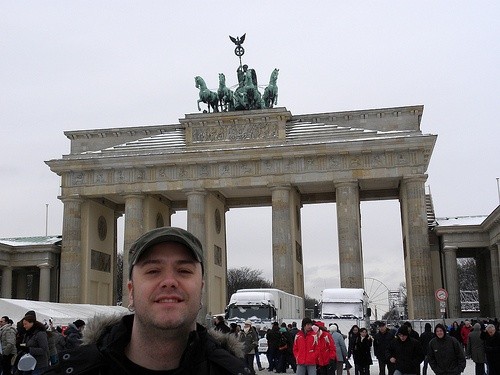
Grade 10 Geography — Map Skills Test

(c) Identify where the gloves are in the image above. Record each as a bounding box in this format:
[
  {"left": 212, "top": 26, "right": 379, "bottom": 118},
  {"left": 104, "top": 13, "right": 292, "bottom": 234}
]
[{"left": 19, "top": 346, "right": 29, "bottom": 353}]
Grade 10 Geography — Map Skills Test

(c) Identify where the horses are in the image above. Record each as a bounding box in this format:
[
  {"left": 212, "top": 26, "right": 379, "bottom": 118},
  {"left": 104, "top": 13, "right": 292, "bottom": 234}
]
[
  {"left": 262, "top": 68, "right": 280, "bottom": 107},
  {"left": 194, "top": 75, "right": 219, "bottom": 114},
  {"left": 242, "top": 69, "right": 260, "bottom": 109},
  {"left": 217, "top": 73, "right": 233, "bottom": 109}
]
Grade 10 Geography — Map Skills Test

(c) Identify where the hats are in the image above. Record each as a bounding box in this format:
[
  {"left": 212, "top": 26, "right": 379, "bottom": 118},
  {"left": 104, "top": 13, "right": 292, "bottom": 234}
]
[
  {"left": 73, "top": 320, "right": 85, "bottom": 328},
  {"left": 128, "top": 227, "right": 204, "bottom": 280},
  {"left": 245, "top": 320, "right": 252, "bottom": 326}
]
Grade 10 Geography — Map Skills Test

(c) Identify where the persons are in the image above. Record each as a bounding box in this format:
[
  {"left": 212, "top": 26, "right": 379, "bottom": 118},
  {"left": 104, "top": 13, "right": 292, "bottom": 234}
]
[
  {"left": 449, "top": 318, "right": 500, "bottom": 375},
  {"left": 237, "top": 64, "right": 249, "bottom": 88},
  {"left": 215, "top": 316, "right": 265, "bottom": 375},
  {"left": 311, "top": 320, "right": 347, "bottom": 375},
  {"left": 293, "top": 317, "right": 320, "bottom": 375},
  {"left": 0, "top": 310, "right": 85, "bottom": 375},
  {"left": 42, "top": 226, "right": 254, "bottom": 375},
  {"left": 347, "top": 325, "right": 373, "bottom": 375},
  {"left": 265, "top": 321, "right": 300, "bottom": 373},
  {"left": 373, "top": 321, "right": 466, "bottom": 375}
]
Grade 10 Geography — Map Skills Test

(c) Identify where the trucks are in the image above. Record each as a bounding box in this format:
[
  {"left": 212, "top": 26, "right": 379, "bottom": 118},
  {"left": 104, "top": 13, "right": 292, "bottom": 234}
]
[
  {"left": 313, "top": 288, "right": 372, "bottom": 351},
  {"left": 225, "top": 288, "right": 304, "bottom": 355}
]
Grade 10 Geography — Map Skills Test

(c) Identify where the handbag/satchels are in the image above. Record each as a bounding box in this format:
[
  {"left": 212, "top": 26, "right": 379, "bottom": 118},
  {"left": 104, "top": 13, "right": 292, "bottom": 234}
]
[
  {"left": 343, "top": 360, "right": 352, "bottom": 369},
  {"left": 18, "top": 354, "right": 37, "bottom": 372}
]
